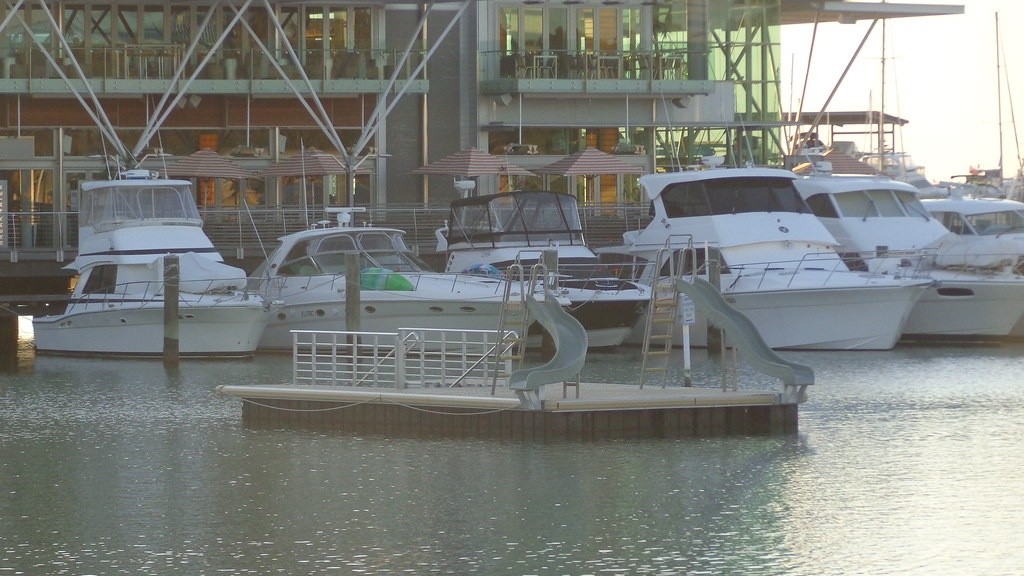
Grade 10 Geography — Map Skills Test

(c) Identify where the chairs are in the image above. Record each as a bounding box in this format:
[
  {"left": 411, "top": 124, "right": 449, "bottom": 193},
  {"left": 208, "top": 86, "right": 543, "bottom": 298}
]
[{"left": 502, "top": 52, "right": 686, "bottom": 81}]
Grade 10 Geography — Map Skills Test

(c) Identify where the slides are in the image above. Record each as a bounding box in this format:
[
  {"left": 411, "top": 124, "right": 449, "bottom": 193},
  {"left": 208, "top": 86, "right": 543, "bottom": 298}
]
[
  {"left": 674, "top": 275, "right": 816, "bottom": 386},
  {"left": 507, "top": 291, "right": 588, "bottom": 391}
]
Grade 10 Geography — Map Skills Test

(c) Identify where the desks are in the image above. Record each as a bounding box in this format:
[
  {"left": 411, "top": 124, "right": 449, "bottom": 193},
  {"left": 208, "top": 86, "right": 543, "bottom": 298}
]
[
  {"left": 510, "top": 55, "right": 557, "bottom": 79},
  {"left": 570, "top": 56, "right": 620, "bottom": 80},
  {"left": 628, "top": 56, "right": 683, "bottom": 80}
]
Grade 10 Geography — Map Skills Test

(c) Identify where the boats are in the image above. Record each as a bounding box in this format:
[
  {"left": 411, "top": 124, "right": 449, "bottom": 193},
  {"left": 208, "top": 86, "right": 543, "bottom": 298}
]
[
  {"left": 918, "top": 198, "right": 1023, "bottom": 257},
  {"left": 792, "top": 160, "right": 1024, "bottom": 344},
  {"left": 594, "top": 154, "right": 936, "bottom": 350},
  {"left": 249, "top": 224, "right": 572, "bottom": 361},
  {"left": 29, "top": 165, "right": 267, "bottom": 359},
  {"left": 440, "top": 189, "right": 655, "bottom": 356}
]
